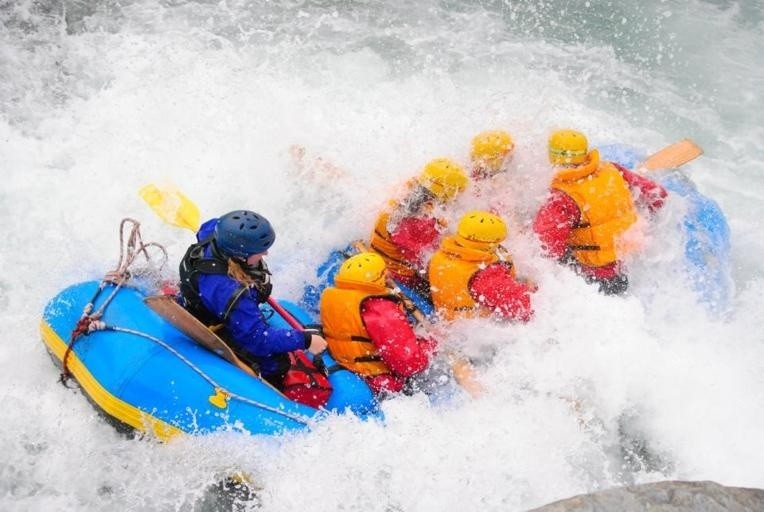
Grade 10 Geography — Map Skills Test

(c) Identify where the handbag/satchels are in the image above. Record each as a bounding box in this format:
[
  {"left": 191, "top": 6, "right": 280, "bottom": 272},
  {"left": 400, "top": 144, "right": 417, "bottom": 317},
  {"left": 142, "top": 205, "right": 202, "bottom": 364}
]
[
  {"left": 281, "top": 350, "right": 333, "bottom": 409},
  {"left": 601, "top": 275, "right": 628, "bottom": 295}
]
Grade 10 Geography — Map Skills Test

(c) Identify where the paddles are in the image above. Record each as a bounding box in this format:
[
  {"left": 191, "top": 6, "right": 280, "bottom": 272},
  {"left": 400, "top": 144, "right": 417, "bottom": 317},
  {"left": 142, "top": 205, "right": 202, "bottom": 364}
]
[
  {"left": 633, "top": 140, "right": 703, "bottom": 175},
  {"left": 351, "top": 241, "right": 491, "bottom": 399},
  {"left": 145, "top": 295, "right": 293, "bottom": 405},
  {"left": 139, "top": 177, "right": 304, "bottom": 331}
]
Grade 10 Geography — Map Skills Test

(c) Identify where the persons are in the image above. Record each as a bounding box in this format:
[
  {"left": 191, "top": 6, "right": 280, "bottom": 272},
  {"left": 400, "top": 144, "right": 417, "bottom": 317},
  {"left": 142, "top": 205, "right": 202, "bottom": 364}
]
[
  {"left": 372, "top": 160, "right": 469, "bottom": 298},
  {"left": 527, "top": 126, "right": 670, "bottom": 296},
  {"left": 460, "top": 128, "right": 540, "bottom": 239},
  {"left": 414, "top": 207, "right": 533, "bottom": 327},
  {"left": 178, "top": 209, "right": 327, "bottom": 385},
  {"left": 318, "top": 254, "right": 436, "bottom": 399}
]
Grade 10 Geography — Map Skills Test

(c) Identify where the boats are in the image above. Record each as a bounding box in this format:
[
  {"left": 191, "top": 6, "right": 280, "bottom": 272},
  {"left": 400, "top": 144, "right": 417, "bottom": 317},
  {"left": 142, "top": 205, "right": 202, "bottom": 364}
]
[{"left": 40, "top": 144, "right": 730, "bottom": 501}]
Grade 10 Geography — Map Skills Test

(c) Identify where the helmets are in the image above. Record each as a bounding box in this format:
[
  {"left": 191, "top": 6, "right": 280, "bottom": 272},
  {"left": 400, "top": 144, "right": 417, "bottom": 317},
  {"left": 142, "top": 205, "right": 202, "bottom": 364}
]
[
  {"left": 213, "top": 210, "right": 276, "bottom": 259},
  {"left": 458, "top": 207, "right": 507, "bottom": 243},
  {"left": 470, "top": 132, "right": 514, "bottom": 171},
  {"left": 547, "top": 130, "right": 587, "bottom": 165},
  {"left": 417, "top": 159, "right": 468, "bottom": 199},
  {"left": 339, "top": 252, "right": 388, "bottom": 289}
]
